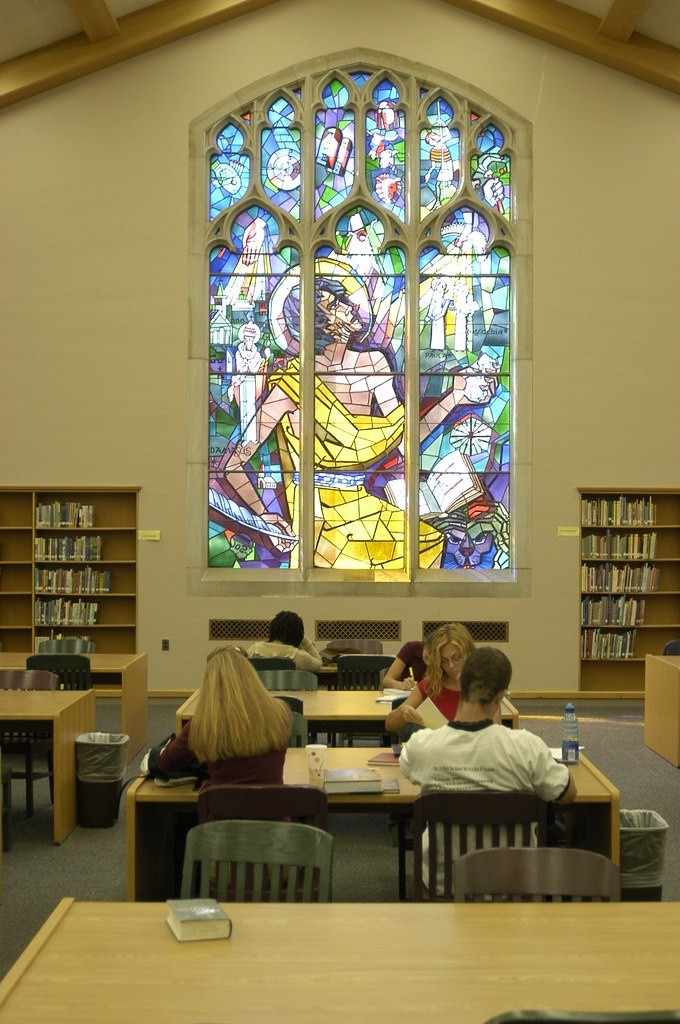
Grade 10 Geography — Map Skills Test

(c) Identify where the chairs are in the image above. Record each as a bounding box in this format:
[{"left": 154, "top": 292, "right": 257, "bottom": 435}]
[
  {"left": 0, "top": 639, "right": 95, "bottom": 853},
  {"left": 181, "top": 640, "right": 621, "bottom": 903},
  {"left": 662, "top": 639, "right": 680, "bottom": 656}
]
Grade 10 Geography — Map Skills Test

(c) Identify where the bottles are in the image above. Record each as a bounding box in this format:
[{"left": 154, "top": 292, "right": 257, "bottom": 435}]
[{"left": 562, "top": 703, "right": 578, "bottom": 764}]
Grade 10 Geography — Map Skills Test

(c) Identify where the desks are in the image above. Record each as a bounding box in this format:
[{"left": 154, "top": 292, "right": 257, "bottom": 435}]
[
  {"left": 0, "top": 688, "right": 95, "bottom": 845},
  {"left": 126, "top": 748, "right": 620, "bottom": 902},
  {"left": 645, "top": 653, "right": 680, "bottom": 768},
  {"left": 176, "top": 690, "right": 520, "bottom": 736},
  {"left": 1, "top": 897, "right": 680, "bottom": 1024},
  {"left": 0, "top": 652, "right": 148, "bottom": 763}
]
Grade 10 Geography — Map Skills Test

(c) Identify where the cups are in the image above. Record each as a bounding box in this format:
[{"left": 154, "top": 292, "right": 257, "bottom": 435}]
[{"left": 305, "top": 744, "right": 327, "bottom": 779}]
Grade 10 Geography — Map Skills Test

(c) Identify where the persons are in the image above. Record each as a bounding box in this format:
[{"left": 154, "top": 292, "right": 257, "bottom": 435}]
[
  {"left": 385, "top": 623, "right": 578, "bottom": 902},
  {"left": 247, "top": 611, "right": 324, "bottom": 673},
  {"left": 161, "top": 646, "right": 296, "bottom": 890}
]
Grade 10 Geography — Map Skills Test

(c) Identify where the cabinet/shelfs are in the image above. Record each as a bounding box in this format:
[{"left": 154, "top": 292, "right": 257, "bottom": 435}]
[
  {"left": 577, "top": 488, "right": 680, "bottom": 691},
  {"left": 0, "top": 486, "right": 142, "bottom": 689}
]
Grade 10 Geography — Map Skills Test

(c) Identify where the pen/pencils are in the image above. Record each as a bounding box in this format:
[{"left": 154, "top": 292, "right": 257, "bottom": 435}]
[{"left": 409, "top": 667, "right": 414, "bottom": 678}]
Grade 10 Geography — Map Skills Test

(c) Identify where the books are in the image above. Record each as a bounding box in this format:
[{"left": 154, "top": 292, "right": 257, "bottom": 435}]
[
  {"left": 375, "top": 686, "right": 412, "bottom": 703},
  {"left": 33, "top": 500, "right": 110, "bottom": 654},
  {"left": 167, "top": 898, "right": 233, "bottom": 941},
  {"left": 581, "top": 495, "right": 661, "bottom": 659},
  {"left": 322, "top": 753, "right": 401, "bottom": 796}
]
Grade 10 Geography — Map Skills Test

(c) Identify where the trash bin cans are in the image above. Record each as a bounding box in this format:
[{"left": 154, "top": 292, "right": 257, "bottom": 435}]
[
  {"left": 75, "top": 733, "right": 129, "bottom": 828},
  {"left": 620, "top": 810, "right": 669, "bottom": 901}
]
